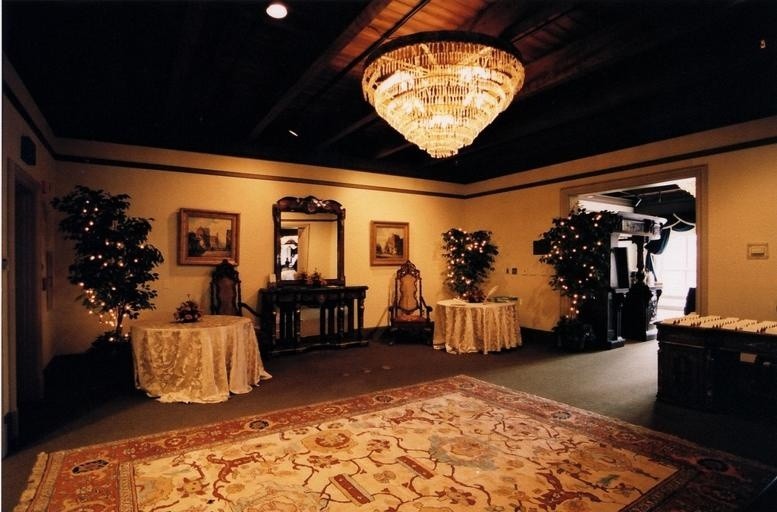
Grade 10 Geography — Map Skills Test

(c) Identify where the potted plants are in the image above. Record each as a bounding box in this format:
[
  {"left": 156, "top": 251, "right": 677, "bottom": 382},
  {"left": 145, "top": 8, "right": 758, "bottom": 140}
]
[
  {"left": 539, "top": 208, "right": 619, "bottom": 352},
  {"left": 46, "top": 184, "right": 164, "bottom": 362}
]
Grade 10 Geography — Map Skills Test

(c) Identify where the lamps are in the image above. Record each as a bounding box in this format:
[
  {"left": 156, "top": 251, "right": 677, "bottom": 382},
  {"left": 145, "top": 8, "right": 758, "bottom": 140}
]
[{"left": 361, "top": 31, "right": 525, "bottom": 160}]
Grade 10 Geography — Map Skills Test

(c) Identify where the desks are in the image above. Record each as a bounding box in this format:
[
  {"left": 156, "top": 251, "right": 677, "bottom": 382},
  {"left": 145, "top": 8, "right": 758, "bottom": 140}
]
[
  {"left": 132, "top": 315, "right": 272, "bottom": 404},
  {"left": 261, "top": 284, "right": 369, "bottom": 357},
  {"left": 433, "top": 299, "right": 522, "bottom": 356},
  {"left": 653, "top": 320, "right": 777, "bottom": 418}
]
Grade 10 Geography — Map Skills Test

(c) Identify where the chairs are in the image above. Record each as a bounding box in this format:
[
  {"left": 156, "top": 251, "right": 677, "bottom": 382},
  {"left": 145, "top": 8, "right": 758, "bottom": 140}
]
[
  {"left": 209, "top": 259, "right": 267, "bottom": 357},
  {"left": 387, "top": 259, "right": 434, "bottom": 346}
]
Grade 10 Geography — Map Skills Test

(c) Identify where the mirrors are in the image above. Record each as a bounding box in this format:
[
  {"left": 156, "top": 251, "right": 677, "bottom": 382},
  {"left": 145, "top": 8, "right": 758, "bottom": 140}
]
[{"left": 272, "top": 196, "right": 345, "bottom": 287}]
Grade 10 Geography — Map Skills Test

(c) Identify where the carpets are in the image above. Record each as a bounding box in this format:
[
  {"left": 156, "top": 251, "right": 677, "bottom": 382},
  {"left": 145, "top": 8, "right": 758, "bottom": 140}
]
[{"left": 13, "top": 375, "right": 776, "bottom": 512}]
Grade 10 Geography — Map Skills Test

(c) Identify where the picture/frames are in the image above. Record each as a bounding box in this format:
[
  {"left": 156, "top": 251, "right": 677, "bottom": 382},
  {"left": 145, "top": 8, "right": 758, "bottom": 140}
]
[
  {"left": 178, "top": 207, "right": 240, "bottom": 266},
  {"left": 370, "top": 221, "right": 409, "bottom": 266}
]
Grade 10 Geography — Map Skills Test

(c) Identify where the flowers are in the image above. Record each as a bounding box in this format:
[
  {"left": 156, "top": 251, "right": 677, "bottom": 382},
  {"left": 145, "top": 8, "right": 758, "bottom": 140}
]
[
  {"left": 174, "top": 293, "right": 201, "bottom": 322},
  {"left": 294, "top": 265, "right": 308, "bottom": 279},
  {"left": 306, "top": 268, "right": 327, "bottom": 286},
  {"left": 463, "top": 288, "right": 485, "bottom": 303}
]
